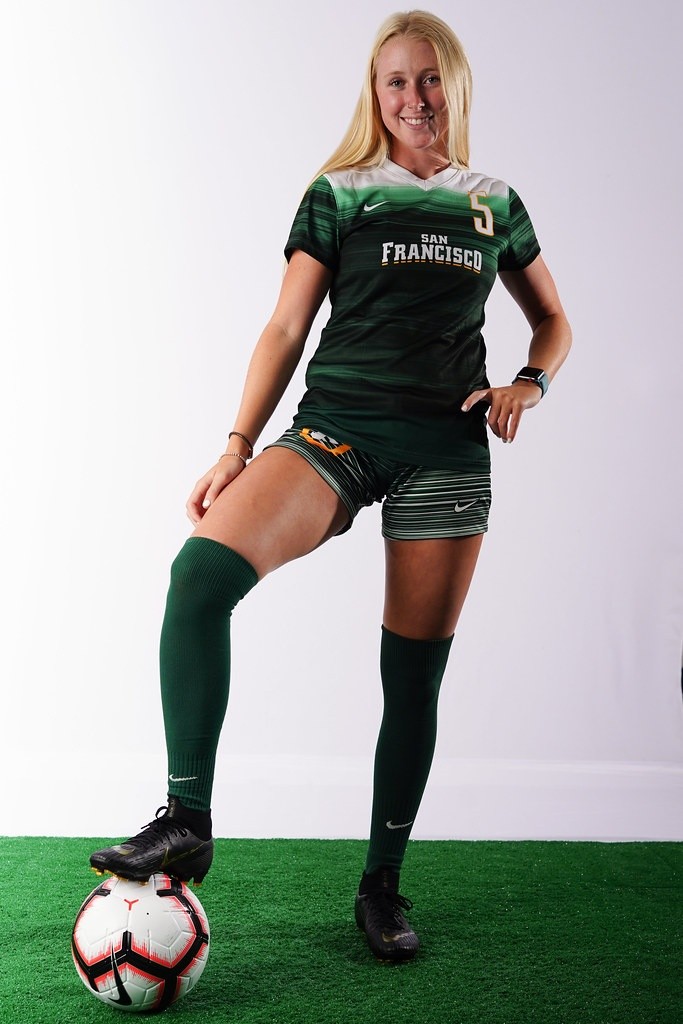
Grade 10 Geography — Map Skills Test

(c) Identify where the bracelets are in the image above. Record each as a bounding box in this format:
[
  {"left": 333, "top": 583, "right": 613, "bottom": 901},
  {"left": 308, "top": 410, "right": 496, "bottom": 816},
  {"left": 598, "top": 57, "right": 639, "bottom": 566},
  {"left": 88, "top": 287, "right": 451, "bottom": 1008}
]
[
  {"left": 228, "top": 432, "right": 254, "bottom": 459},
  {"left": 218, "top": 452, "right": 247, "bottom": 468}
]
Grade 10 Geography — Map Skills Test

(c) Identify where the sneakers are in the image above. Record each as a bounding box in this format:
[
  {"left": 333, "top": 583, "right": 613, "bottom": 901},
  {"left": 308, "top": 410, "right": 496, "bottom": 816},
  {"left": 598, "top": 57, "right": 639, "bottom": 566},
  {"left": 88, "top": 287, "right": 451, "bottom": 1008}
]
[
  {"left": 89, "top": 795, "right": 214, "bottom": 887},
  {"left": 355, "top": 868, "right": 418, "bottom": 959}
]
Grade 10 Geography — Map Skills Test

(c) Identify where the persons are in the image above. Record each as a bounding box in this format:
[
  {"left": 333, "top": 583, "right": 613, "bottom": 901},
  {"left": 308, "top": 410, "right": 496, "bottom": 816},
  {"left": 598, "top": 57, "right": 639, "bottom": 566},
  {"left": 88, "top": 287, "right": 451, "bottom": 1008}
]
[{"left": 83, "top": 10, "right": 574, "bottom": 966}]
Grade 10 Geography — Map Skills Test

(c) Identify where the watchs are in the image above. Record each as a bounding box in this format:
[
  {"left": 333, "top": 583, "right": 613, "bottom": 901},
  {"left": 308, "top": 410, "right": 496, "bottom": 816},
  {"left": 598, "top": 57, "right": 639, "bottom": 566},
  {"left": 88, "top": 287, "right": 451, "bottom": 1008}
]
[{"left": 511, "top": 366, "right": 549, "bottom": 399}]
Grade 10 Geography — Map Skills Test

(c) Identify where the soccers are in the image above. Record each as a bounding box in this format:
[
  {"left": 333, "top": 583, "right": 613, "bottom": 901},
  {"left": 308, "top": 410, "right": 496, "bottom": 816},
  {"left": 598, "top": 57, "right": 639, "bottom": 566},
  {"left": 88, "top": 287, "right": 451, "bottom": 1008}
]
[{"left": 71, "top": 873, "right": 211, "bottom": 1011}]
